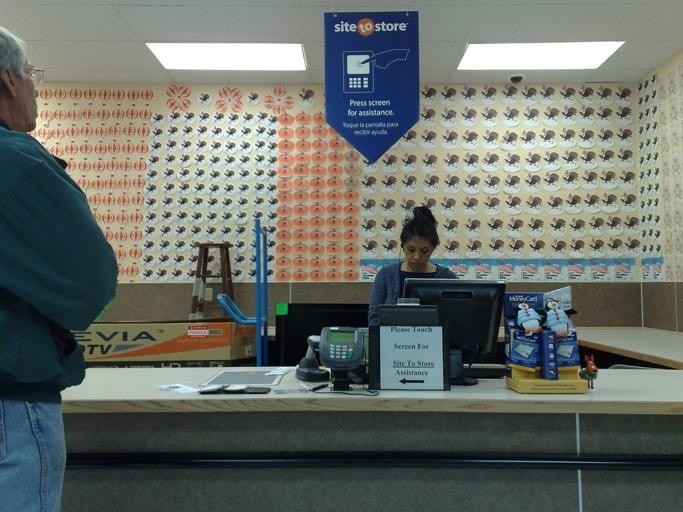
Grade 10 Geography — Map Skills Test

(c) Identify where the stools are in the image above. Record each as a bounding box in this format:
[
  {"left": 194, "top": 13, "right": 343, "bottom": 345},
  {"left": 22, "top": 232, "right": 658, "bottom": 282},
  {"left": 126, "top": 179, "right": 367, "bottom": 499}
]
[{"left": 187, "top": 243, "right": 234, "bottom": 319}]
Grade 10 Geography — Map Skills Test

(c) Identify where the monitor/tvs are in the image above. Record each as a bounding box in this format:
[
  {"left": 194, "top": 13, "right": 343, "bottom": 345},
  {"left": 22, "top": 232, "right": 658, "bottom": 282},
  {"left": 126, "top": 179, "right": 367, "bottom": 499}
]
[{"left": 401, "top": 278, "right": 506, "bottom": 386}]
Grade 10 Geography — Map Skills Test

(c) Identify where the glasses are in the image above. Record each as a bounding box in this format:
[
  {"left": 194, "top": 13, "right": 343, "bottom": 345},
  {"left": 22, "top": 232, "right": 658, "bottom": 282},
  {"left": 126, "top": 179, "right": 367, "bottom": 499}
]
[{"left": 20, "top": 65, "right": 45, "bottom": 83}]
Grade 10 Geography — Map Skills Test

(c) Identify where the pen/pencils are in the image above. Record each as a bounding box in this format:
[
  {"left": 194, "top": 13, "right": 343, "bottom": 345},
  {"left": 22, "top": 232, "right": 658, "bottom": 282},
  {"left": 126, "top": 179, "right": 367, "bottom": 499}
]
[{"left": 312, "top": 383, "right": 328, "bottom": 391}]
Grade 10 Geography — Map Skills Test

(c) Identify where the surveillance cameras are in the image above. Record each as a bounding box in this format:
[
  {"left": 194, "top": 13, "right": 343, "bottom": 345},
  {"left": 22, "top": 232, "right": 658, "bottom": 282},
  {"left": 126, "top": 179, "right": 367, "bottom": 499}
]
[{"left": 508, "top": 73, "right": 524, "bottom": 84}]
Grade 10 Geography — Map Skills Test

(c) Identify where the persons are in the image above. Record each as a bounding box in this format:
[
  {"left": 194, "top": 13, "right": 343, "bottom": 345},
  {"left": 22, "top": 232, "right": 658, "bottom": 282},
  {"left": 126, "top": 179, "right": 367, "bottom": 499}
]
[
  {"left": 0, "top": 28, "right": 120, "bottom": 512},
  {"left": 369, "top": 207, "right": 460, "bottom": 326}
]
